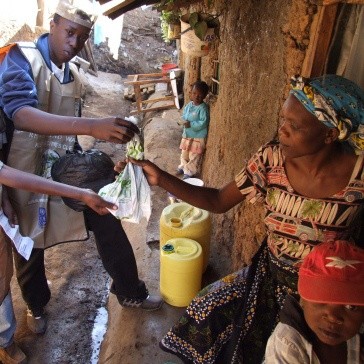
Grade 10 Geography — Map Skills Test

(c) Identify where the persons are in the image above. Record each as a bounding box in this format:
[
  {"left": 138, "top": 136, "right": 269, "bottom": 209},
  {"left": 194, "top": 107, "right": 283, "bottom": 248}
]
[
  {"left": 0, "top": 0, "right": 165, "bottom": 364},
  {"left": 176, "top": 81, "right": 210, "bottom": 179},
  {"left": 262, "top": 240, "right": 364, "bottom": 364},
  {"left": 114, "top": 73, "right": 364, "bottom": 364}
]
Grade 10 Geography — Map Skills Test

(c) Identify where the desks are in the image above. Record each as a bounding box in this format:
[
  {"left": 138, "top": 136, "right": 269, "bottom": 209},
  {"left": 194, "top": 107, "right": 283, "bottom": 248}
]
[{"left": 124, "top": 68, "right": 180, "bottom": 116}]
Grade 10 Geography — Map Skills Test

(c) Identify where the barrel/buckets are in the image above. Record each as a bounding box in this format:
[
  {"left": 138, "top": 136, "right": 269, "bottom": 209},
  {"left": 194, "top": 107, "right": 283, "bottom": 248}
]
[
  {"left": 160, "top": 238, "right": 204, "bottom": 308},
  {"left": 160, "top": 203, "right": 210, "bottom": 275}
]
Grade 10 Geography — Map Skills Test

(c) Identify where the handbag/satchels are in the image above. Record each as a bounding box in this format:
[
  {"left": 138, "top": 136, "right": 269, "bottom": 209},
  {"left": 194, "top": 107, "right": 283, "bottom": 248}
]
[{"left": 51, "top": 149, "right": 116, "bottom": 213}]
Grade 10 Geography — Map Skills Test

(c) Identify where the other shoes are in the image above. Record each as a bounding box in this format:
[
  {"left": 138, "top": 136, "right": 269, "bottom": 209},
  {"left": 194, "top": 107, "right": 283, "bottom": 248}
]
[
  {"left": 0, "top": 341, "right": 28, "bottom": 364},
  {"left": 175, "top": 169, "right": 184, "bottom": 176},
  {"left": 182, "top": 174, "right": 192, "bottom": 179}
]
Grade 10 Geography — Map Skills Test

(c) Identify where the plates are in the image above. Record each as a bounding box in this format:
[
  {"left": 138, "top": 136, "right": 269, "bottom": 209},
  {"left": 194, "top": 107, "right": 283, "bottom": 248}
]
[{"left": 182, "top": 177, "right": 205, "bottom": 187}]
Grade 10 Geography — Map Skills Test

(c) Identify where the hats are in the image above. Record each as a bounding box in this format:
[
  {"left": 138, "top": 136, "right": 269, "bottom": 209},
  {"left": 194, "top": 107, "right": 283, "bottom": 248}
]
[
  {"left": 48, "top": 0, "right": 99, "bottom": 30},
  {"left": 292, "top": 240, "right": 364, "bottom": 306},
  {"left": 288, "top": 73, "right": 364, "bottom": 139}
]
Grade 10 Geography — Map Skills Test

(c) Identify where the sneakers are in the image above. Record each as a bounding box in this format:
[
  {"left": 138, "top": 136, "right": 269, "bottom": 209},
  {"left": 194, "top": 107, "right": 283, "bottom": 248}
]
[
  {"left": 26, "top": 306, "right": 47, "bottom": 336},
  {"left": 117, "top": 294, "right": 165, "bottom": 311}
]
